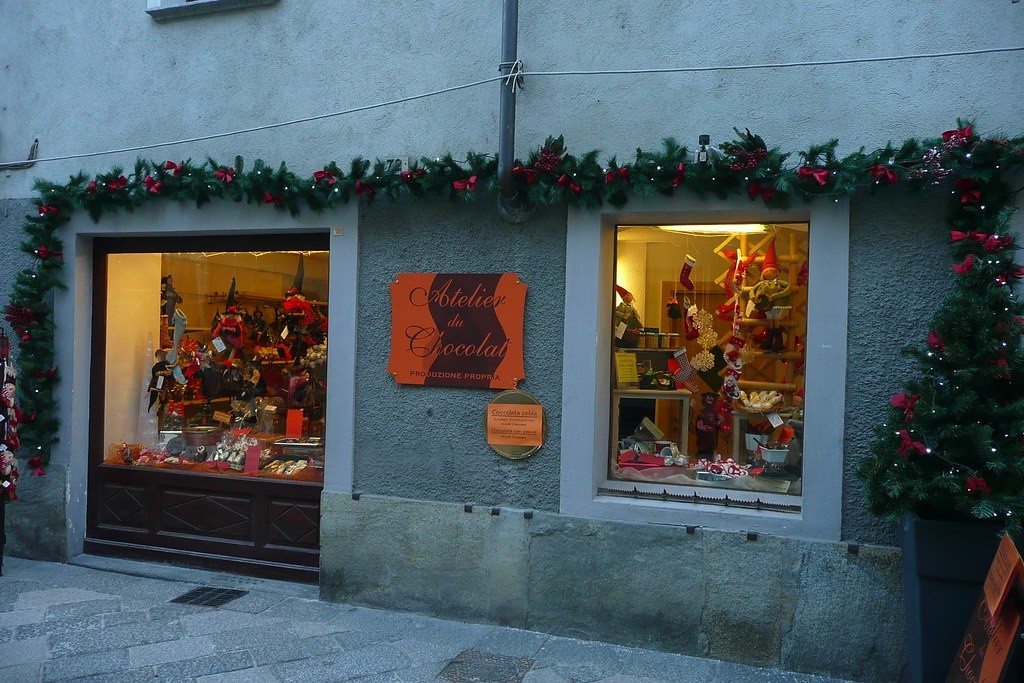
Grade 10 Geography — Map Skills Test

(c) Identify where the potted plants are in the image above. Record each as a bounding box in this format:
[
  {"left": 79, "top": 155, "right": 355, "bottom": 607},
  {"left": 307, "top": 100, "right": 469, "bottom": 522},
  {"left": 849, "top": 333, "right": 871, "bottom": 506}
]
[{"left": 856, "top": 260, "right": 1024, "bottom": 683}]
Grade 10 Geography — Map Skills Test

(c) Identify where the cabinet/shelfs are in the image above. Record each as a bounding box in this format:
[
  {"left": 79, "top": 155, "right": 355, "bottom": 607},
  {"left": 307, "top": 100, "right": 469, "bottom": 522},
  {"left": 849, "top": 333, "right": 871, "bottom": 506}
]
[
  {"left": 613, "top": 347, "right": 692, "bottom": 462},
  {"left": 161, "top": 289, "right": 329, "bottom": 430}
]
